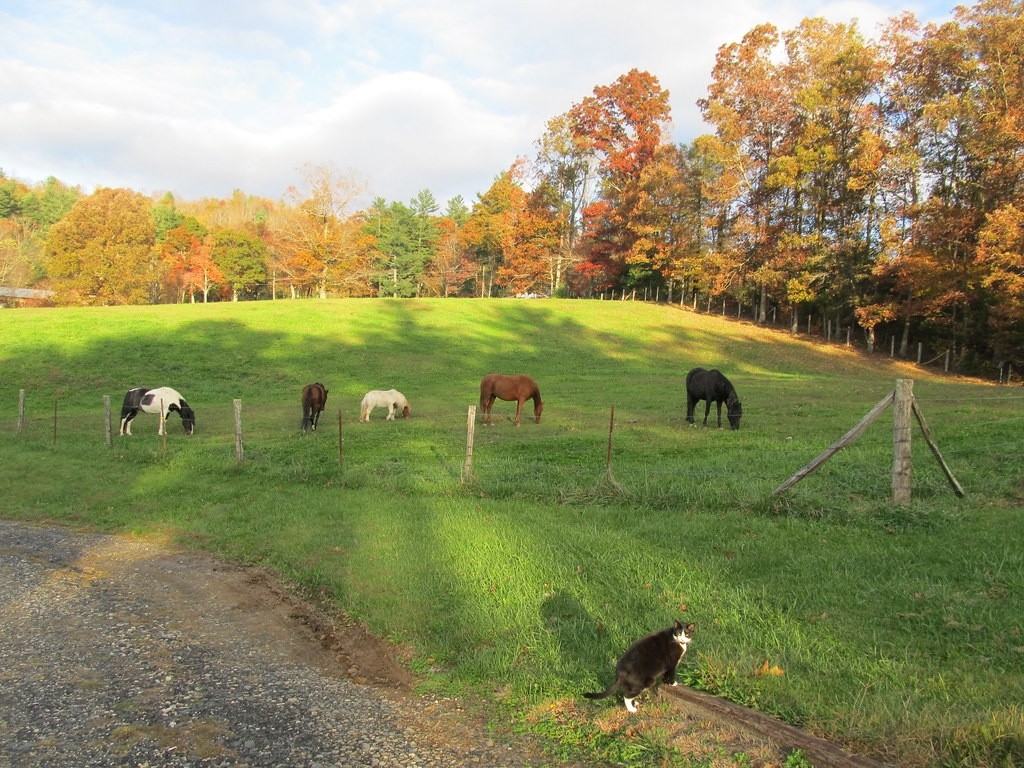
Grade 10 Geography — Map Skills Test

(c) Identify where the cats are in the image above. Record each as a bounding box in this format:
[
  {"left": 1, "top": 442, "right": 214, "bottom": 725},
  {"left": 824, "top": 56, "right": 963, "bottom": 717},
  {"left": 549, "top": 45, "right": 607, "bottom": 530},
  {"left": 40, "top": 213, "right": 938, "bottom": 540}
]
[{"left": 581, "top": 618, "right": 697, "bottom": 713}]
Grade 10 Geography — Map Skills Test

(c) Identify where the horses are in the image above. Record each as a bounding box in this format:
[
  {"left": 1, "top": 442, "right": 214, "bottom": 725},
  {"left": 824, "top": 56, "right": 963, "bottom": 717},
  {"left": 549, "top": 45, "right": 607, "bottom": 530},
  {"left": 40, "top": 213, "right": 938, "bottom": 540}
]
[
  {"left": 356, "top": 388, "right": 411, "bottom": 424},
  {"left": 684, "top": 368, "right": 743, "bottom": 431},
  {"left": 118, "top": 386, "right": 196, "bottom": 438},
  {"left": 300, "top": 382, "right": 329, "bottom": 434},
  {"left": 479, "top": 373, "right": 544, "bottom": 427}
]
